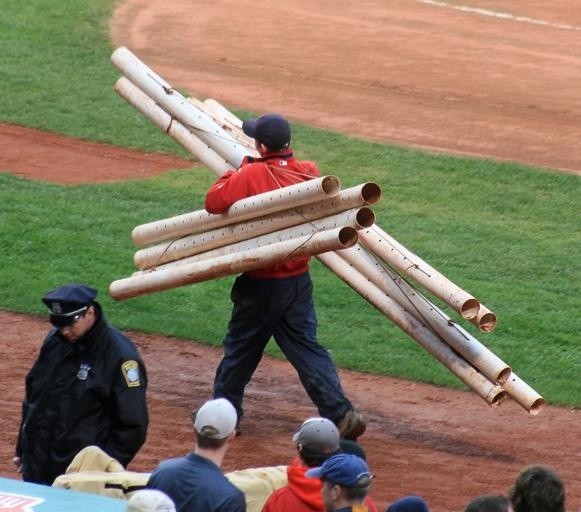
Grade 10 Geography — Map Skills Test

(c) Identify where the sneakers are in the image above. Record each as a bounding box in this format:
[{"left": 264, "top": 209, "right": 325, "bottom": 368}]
[{"left": 337, "top": 409, "right": 367, "bottom": 442}]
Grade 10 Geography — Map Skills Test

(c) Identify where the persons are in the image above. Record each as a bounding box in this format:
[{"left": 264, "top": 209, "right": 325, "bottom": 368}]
[
  {"left": 189, "top": 113, "right": 367, "bottom": 443},
  {"left": 12, "top": 283, "right": 150, "bottom": 487}
]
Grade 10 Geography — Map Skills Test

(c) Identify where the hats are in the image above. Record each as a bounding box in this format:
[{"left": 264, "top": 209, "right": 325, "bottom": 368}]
[
  {"left": 125, "top": 488, "right": 179, "bottom": 512},
  {"left": 241, "top": 112, "right": 292, "bottom": 150},
  {"left": 292, "top": 415, "right": 342, "bottom": 455},
  {"left": 192, "top": 396, "right": 240, "bottom": 442},
  {"left": 40, "top": 283, "right": 99, "bottom": 328},
  {"left": 303, "top": 453, "right": 376, "bottom": 491}
]
